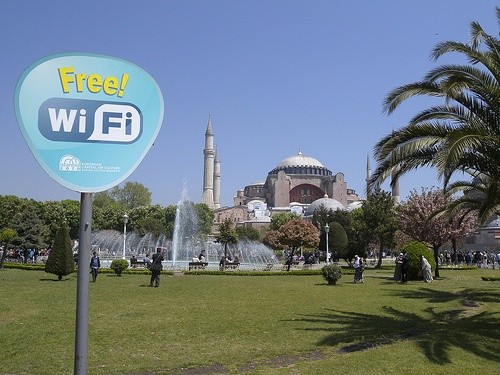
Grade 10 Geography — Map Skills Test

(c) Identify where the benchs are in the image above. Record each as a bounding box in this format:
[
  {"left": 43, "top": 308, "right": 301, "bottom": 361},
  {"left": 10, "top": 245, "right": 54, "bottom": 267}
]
[
  {"left": 188, "top": 262, "right": 209, "bottom": 271},
  {"left": 131, "top": 261, "right": 152, "bottom": 268},
  {"left": 219, "top": 262, "right": 241, "bottom": 271},
  {"left": 303, "top": 261, "right": 316, "bottom": 265},
  {"left": 282, "top": 260, "right": 300, "bottom": 269}
]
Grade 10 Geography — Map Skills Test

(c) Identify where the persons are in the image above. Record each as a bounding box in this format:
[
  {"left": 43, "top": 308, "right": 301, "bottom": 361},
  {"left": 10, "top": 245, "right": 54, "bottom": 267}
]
[
  {"left": 394, "top": 250, "right": 409, "bottom": 283},
  {"left": 0, "top": 247, "right": 38, "bottom": 263},
  {"left": 130, "top": 256, "right": 137, "bottom": 267},
  {"left": 192, "top": 255, "right": 200, "bottom": 270},
  {"left": 419, "top": 254, "right": 431, "bottom": 283},
  {"left": 73, "top": 245, "right": 80, "bottom": 265},
  {"left": 199, "top": 250, "right": 205, "bottom": 270},
  {"left": 149, "top": 248, "right": 164, "bottom": 287},
  {"left": 219, "top": 257, "right": 225, "bottom": 270},
  {"left": 351, "top": 254, "right": 366, "bottom": 284},
  {"left": 143, "top": 254, "right": 151, "bottom": 265},
  {"left": 227, "top": 256, "right": 240, "bottom": 270},
  {"left": 90, "top": 251, "right": 101, "bottom": 282},
  {"left": 284, "top": 247, "right": 331, "bottom": 271},
  {"left": 435, "top": 249, "right": 500, "bottom": 270}
]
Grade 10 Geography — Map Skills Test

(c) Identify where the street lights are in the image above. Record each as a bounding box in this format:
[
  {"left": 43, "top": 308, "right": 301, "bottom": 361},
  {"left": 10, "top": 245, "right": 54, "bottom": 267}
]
[
  {"left": 122, "top": 212, "right": 129, "bottom": 259},
  {"left": 324, "top": 223, "right": 330, "bottom": 263}
]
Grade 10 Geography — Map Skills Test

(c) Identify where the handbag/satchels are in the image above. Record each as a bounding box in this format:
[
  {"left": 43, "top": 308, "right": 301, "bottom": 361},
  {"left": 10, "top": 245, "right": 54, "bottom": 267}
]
[{"left": 147, "top": 263, "right": 154, "bottom": 272}]
